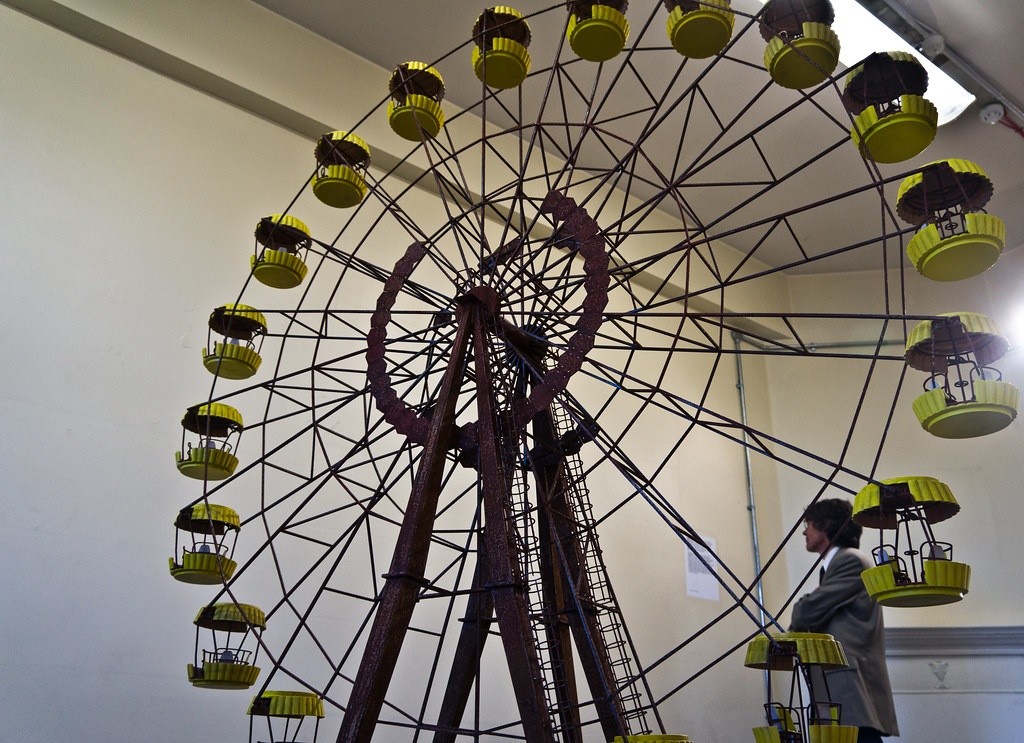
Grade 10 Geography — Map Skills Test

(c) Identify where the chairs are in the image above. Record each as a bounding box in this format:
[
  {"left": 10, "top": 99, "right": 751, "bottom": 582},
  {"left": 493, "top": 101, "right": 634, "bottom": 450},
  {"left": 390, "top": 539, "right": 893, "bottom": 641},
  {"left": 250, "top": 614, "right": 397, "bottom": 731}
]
[
  {"left": 925, "top": 546, "right": 949, "bottom": 561},
  {"left": 810, "top": 705, "right": 833, "bottom": 726},
  {"left": 767, "top": 706, "right": 800, "bottom": 734},
  {"left": 217, "top": 650, "right": 235, "bottom": 663},
  {"left": 976, "top": 372, "right": 994, "bottom": 382},
  {"left": 876, "top": 546, "right": 900, "bottom": 579},
  {"left": 928, "top": 379, "right": 959, "bottom": 405},
  {"left": 195, "top": 544, "right": 211, "bottom": 553}
]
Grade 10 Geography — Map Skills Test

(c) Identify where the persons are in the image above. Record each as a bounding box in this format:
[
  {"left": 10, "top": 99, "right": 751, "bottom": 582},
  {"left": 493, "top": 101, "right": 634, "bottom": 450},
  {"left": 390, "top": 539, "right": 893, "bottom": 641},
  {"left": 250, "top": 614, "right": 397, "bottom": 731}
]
[{"left": 788, "top": 498, "right": 901, "bottom": 743}]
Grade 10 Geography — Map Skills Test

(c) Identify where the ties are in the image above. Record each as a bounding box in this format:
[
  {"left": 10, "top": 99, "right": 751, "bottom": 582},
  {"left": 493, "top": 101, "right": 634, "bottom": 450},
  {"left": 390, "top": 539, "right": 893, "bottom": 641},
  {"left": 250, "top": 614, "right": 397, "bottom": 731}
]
[{"left": 819, "top": 565, "right": 826, "bottom": 586}]
